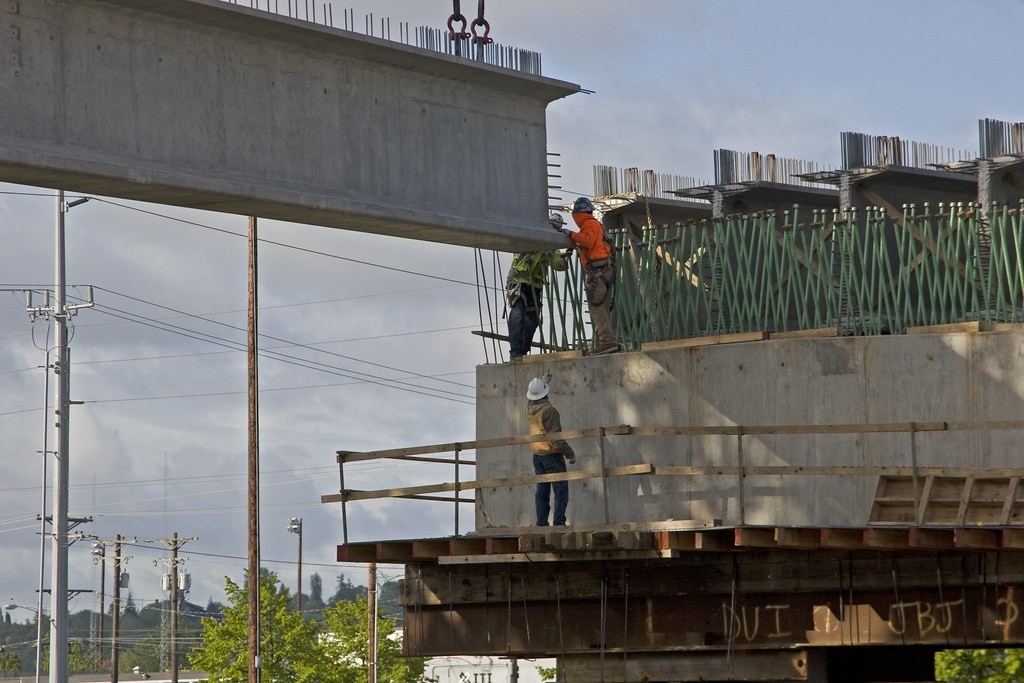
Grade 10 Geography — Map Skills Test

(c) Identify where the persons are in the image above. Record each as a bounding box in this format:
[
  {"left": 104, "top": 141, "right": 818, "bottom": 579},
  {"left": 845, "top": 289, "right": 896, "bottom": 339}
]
[
  {"left": 505, "top": 213, "right": 569, "bottom": 362},
  {"left": 524, "top": 377, "right": 577, "bottom": 526},
  {"left": 562, "top": 197, "right": 620, "bottom": 357}
]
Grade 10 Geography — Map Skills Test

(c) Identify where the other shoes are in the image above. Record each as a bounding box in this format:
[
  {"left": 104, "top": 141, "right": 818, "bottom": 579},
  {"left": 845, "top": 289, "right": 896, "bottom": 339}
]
[
  {"left": 590, "top": 343, "right": 618, "bottom": 355},
  {"left": 510, "top": 353, "right": 527, "bottom": 361}
]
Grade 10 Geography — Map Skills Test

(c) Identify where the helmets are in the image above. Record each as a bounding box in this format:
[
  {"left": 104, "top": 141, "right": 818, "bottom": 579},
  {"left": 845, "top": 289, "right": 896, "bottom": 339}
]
[
  {"left": 549, "top": 213, "right": 563, "bottom": 228},
  {"left": 573, "top": 197, "right": 596, "bottom": 212},
  {"left": 527, "top": 378, "right": 549, "bottom": 400}
]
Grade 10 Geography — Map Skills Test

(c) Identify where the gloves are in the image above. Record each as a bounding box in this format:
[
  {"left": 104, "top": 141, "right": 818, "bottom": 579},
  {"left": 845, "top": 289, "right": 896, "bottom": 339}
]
[
  {"left": 542, "top": 369, "right": 552, "bottom": 383},
  {"left": 569, "top": 457, "right": 575, "bottom": 464},
  {"left": 558, "top": 229, "right": 570, "bottom": 234}
]
